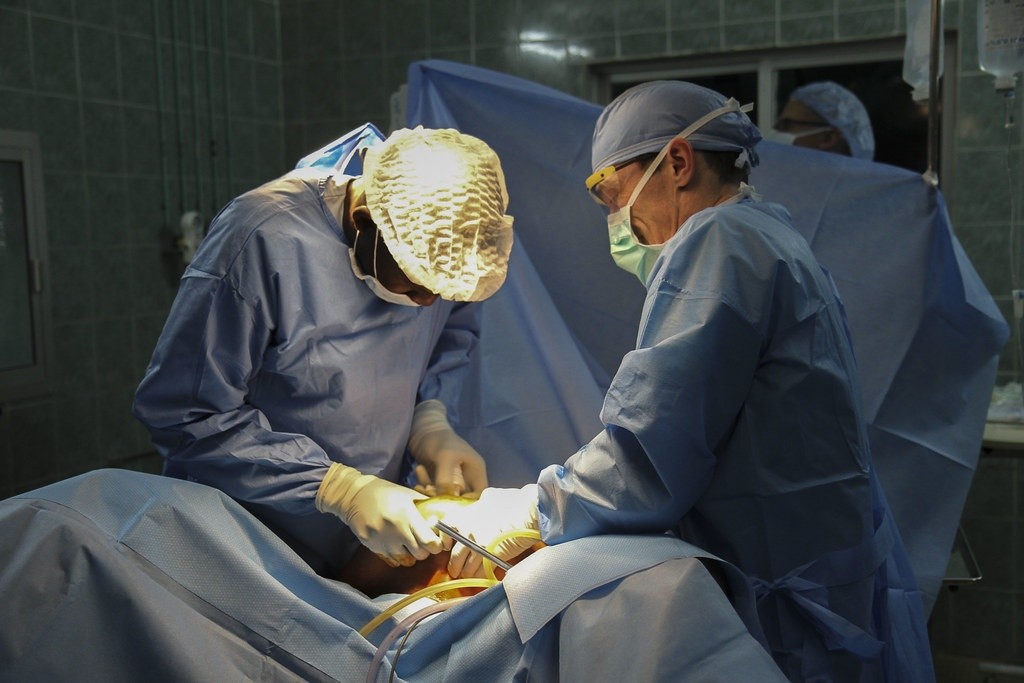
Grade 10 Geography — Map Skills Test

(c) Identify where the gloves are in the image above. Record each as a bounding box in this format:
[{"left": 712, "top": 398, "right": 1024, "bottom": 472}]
[
  {"left": 430, "top": 484, "right": 541, "bottom": 579},
  {"left": 406, "top": 400, "right": 489, "bottom": 500},
  {"left": 315, "top": 461, "right": 453, "bottom": 568}
]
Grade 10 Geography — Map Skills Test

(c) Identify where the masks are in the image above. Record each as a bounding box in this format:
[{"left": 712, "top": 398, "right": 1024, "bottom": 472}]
[
  {"left": 764, "top": 128, "right": 834, "bottom": 147},
  {"left": 350, "top": 224, "right": 422, "bottom": 307},
  {"left": 607, "top": 146, "right": 677, "bottom": 288}
]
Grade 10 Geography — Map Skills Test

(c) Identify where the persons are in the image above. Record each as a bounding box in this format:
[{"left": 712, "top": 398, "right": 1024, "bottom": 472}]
[
  {"left": 767, "top": 82, "right": 875, "bottom": 160},
  {"left": 438, "top": 80, "right": 936, "bottom": 683},
  {"left": 130, "top": 123, "right": 513, "bottom": 579}
]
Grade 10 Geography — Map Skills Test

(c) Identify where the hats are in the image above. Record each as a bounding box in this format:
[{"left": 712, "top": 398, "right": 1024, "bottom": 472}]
[
  {"left": 592, "top": 79, "right": 762, "bottom": 173},
  {"left": 362, "top": 126, "right": 515, "bottom": 303}
]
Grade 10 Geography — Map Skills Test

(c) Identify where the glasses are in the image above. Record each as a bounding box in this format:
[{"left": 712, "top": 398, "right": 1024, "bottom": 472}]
[{"left": 774, "top": 118, "right": 830, "bottom": 133}]
